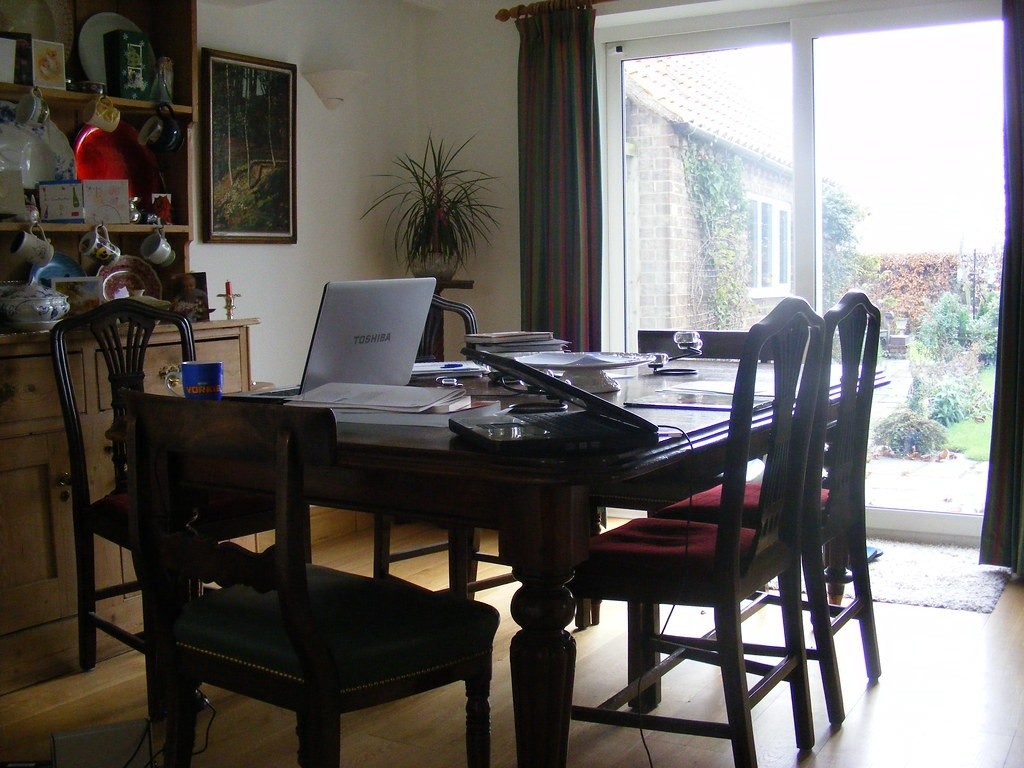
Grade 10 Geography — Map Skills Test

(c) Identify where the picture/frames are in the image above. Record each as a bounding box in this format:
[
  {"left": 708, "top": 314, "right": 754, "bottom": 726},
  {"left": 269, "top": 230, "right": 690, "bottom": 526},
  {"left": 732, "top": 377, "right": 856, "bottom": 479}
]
[{"left": 201, "top": 47, "right": 296, "bottom": 244}]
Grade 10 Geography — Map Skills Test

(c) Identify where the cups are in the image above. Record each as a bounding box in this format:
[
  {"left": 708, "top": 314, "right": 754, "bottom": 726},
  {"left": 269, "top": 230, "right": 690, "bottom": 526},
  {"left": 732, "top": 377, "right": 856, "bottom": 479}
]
[
  {"left": 140, "top": 226, "right": 175, "bottom": 266},
  {"left": 80, "top": 81, "right": 106, "bottom": 95},
  {"left": 13, "top": 223, "right": 54, "bottom": 266},
  {"left": 78, "top": 223, "right": 120, "bottom": 268},
  {"left": 165, "top": 362, "right": 225, "bottom": 399},
  {"left": 137, "top": 103, "right": 184, "bottom": 155},
  {"left": 16, "top": 87, "right": 50, "bottom": 124},
  {"left": 81, "top": 95, "right": 121, "bottom": 131}
]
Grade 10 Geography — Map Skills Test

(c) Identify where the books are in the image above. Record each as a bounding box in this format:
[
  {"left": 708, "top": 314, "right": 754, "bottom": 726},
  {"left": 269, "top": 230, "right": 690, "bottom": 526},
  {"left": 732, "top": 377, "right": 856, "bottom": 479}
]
[
  {"left": 410, "top": 360, "right": 487, "bottom": 381},
  {"left": 285, "top": 381, "right": 503, "bottom": 428},
  {"left": 463, "top": 330, "right": 573, "bottom": 376}
]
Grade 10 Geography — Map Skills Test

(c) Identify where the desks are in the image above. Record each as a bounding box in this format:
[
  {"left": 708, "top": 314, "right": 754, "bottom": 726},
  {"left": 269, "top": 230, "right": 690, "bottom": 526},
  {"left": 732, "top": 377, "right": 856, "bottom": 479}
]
[{"left": 191, "top": 349, "right": 890, "bottom": 766}]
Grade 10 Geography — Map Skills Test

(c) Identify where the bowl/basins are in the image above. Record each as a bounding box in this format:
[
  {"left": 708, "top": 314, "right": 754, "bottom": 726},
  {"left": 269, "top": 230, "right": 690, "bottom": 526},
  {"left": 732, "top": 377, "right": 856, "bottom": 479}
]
[{"left": 0, "top": 284, "right": 70, "bottom": 321}]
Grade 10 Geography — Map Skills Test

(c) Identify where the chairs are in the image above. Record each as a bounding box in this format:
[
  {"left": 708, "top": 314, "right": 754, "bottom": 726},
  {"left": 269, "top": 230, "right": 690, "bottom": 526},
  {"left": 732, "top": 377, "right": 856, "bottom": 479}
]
[
  {"left": 658, "top": 294, "right": 886, "bottom": 728},
  {"left": 118, "top": 385, "right": 500, "bottom": 768},
  {"left": 48, "top": 299, "right": 279, "bottom": 724},
  {"left": 572, "top": 298, "right": 830, "bottom": 766},
  {"left": 371, "top": 294, "right": 591, "bottom": 629}
]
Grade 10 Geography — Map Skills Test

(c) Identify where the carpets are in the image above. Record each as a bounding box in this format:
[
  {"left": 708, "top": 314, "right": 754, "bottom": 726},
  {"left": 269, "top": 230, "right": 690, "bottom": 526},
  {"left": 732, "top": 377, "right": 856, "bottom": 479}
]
[{"left": 766, "top": 536, "right": 1012, "bottom": 613}]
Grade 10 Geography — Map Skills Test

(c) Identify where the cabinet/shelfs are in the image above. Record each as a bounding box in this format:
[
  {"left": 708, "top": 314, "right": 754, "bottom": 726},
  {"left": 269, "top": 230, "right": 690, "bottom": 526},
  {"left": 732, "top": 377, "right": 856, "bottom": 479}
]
[{"left": 1, "top": 0, "right": 260, "bottom": 694}]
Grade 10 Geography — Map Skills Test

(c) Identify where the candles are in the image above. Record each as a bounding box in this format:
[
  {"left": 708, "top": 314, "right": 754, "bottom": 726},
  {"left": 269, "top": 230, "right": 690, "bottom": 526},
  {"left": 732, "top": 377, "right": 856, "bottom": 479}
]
[{"left": 226, "top": 280, "right": 232, "bottom": 295}]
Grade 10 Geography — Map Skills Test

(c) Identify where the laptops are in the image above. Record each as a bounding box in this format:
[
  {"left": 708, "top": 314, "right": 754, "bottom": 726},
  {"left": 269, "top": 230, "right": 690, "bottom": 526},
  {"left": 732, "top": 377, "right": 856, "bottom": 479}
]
[
  {"left": 449, "top": 347, "right": 659, "bottom": 454},
  {"left": 220, "top": 277, "right": 437, "bottom": 403}
]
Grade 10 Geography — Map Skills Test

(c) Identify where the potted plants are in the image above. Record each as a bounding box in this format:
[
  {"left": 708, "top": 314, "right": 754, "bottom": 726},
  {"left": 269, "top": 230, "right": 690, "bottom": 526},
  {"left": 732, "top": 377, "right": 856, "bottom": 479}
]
[{"left": 359, "top": 133, "right": 506, "bottom": 282}]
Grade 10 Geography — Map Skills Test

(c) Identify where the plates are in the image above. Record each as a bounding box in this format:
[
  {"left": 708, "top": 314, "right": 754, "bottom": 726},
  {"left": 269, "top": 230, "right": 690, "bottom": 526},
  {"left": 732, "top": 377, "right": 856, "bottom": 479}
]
[
  {"left": 78, "top": 12, "right": 157, "bottom": 92},
  {"left": 96, "top": 254, "right": 162, "bottom": 301},
  {"left": 0, "top": 0, "right": 73, "bottom": 61},
  {"left": 0, "top": 101, "right": 77, "bottom": 215},
  {"left": 74, "top": 119, "right": 170, "bottom": 221},
  {"left": 29, "top": 253, "right": 88, "bottom": 289},
  {"left": 3, "top": 321, "right": 61, "bottom": 332}
]
